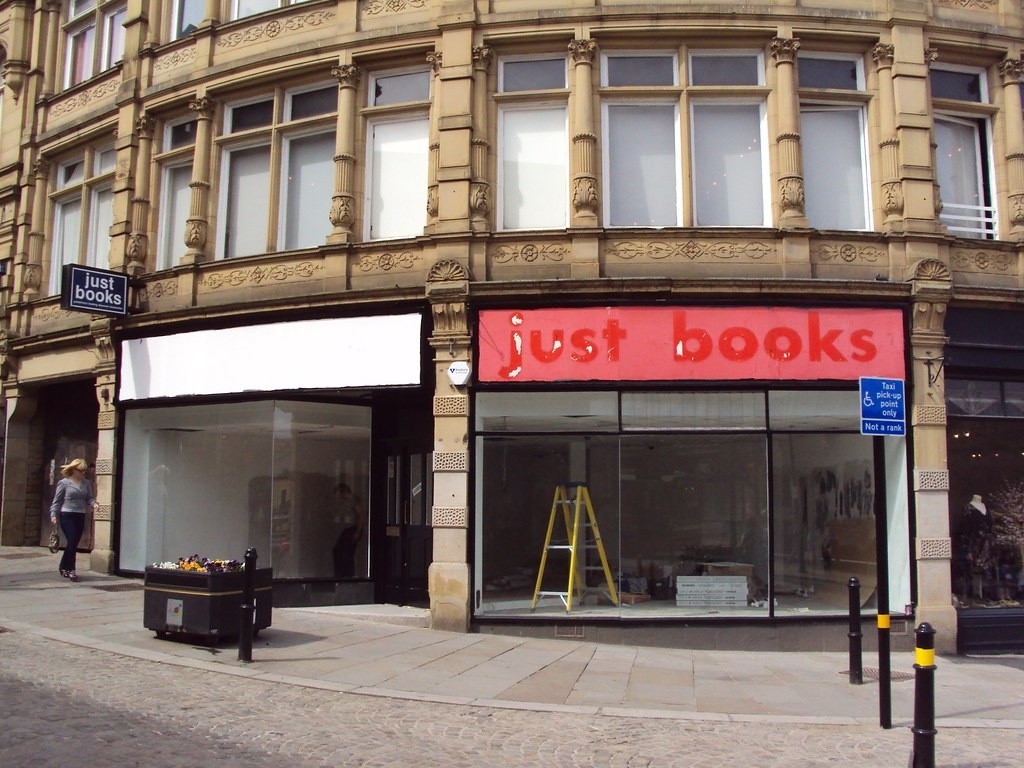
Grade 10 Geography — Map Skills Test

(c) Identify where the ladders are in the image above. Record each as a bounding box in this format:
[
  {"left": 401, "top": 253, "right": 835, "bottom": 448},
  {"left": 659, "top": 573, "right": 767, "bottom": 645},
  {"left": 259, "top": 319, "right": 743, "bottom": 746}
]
[{"left": 531, "top": 481, "right": 621, "bottom": 613}]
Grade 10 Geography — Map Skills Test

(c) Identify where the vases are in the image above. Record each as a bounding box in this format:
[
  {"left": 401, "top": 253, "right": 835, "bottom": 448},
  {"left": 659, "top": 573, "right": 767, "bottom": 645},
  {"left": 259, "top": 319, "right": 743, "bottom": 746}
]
[{"left": 143, "top": 564, "right": 274, "bottom": 646}]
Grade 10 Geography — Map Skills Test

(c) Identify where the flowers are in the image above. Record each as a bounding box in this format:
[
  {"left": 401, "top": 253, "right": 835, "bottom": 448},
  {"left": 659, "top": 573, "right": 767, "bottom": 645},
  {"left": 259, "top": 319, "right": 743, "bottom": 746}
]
[
  {"left": 985, "top": 477, "right": 1024, "bottom": 548},
  {"left": 153, "top": 553, "right": 245, "bottom": 574}
]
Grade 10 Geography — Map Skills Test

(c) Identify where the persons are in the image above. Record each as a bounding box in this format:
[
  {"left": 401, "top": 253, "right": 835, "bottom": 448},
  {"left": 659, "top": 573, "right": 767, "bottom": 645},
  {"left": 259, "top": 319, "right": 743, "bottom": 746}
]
[
  {"left": 49, "top": 458, "right": 100, "bottom": 582},
  {"left": 331, "top": 483, "right": 365, "bottom": 576},
  {"left": 957, "top": 494, "right": 1001, "bottom": 606},
  {"left": 138, "top": 465, "right": 169, "bottom": 563}
]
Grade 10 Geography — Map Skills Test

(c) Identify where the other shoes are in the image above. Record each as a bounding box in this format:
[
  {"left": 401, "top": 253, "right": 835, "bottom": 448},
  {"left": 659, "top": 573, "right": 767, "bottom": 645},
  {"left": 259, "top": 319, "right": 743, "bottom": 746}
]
[{"left": 59, "top": 568, "right": 79, "bottom": 581}]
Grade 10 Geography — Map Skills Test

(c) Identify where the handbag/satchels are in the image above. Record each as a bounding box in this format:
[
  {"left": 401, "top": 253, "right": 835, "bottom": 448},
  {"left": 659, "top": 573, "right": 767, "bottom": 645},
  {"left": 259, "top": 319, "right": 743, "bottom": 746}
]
[{"left": 48, "top": 521, "right": 60, "bottom": 554}]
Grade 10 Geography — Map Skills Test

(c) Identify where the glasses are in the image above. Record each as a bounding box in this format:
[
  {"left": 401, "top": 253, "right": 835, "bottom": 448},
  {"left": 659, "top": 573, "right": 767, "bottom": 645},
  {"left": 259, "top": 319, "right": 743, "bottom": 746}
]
[{"left": 75, "top": 467, "right": 88, "bottom": 472}]
